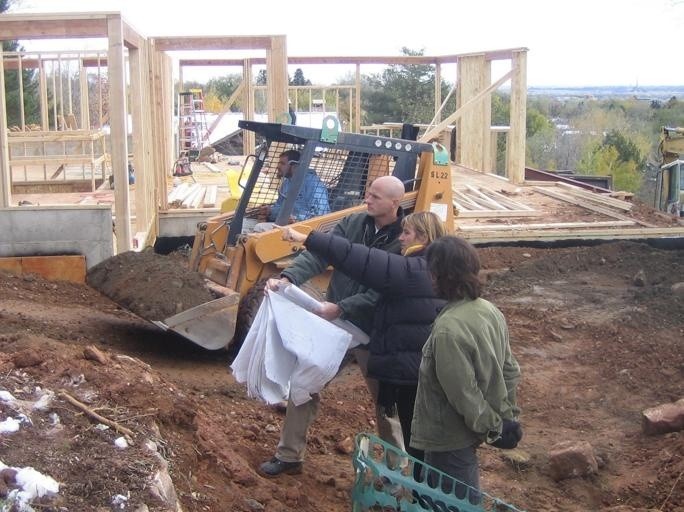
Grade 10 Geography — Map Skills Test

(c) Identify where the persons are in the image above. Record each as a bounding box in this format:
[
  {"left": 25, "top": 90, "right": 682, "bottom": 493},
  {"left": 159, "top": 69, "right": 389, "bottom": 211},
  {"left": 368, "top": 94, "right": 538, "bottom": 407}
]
[
  {"left": 409, "top": 233, "right": 522, "bottom": 511},
  {"left": 271, "top": 210, "right": 449, "bottom": 508},
  {"left": 258, "top": 175, "right": 406, "bottom": 479},
  {"left": 256, "top": 150, "right": 331, "bottom": 224}
]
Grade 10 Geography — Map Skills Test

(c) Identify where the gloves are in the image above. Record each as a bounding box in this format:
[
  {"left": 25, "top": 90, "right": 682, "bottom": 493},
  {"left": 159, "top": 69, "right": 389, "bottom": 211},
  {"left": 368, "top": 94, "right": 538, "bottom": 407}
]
[{"left": 488, "top": 417, "right": 525, "bottom": 449}]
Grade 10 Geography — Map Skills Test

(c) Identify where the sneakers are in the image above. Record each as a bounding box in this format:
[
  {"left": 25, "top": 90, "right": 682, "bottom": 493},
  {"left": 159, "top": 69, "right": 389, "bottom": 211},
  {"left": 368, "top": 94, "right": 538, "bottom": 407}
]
[
  {"left": 259, "top": 453, "right": 304, "bottom": 477},
  {"left": 379, "top": 465, "right": 407, "bottom": 500}
]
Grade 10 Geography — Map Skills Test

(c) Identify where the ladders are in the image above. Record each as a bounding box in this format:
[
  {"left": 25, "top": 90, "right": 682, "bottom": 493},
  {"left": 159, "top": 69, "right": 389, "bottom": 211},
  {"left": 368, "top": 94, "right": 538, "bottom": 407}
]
[{"left": 179, "top": 88, "right": 211, "bottom": 158}]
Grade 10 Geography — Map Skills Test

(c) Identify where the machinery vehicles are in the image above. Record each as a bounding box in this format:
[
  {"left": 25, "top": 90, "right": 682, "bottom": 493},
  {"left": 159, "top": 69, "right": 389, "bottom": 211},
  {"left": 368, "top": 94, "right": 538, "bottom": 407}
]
[
  {"left": 83, "top": 114, "right": 460, "bottom": 352},
  {"left": 652, "top": 125, "right": 684, "bottom": 222}
]
